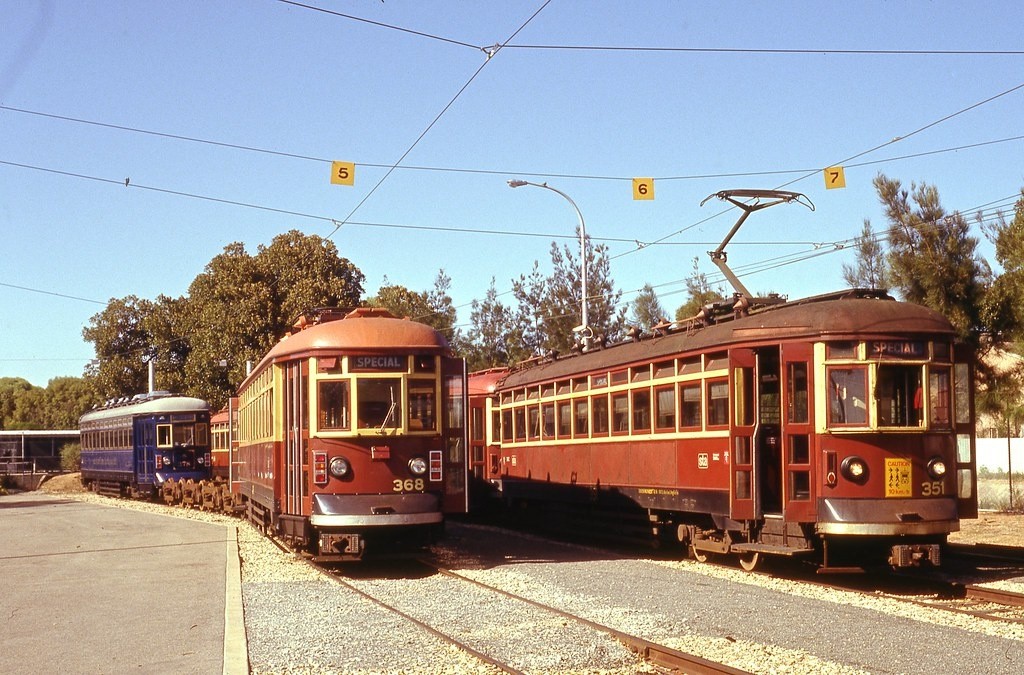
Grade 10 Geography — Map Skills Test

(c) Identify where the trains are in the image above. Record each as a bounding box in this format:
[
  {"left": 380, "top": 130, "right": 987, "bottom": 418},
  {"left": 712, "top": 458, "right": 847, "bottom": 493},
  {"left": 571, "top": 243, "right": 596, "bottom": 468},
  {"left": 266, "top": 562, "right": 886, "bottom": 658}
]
[
  {"left": 229, "top": 305, "right": 469, "bottom": 570},
  {"left": 207, "top": 287, "right": 980, "bottom": 576},
  {"left": 78, "top": 389, "right": 212, "bottom": 502}
]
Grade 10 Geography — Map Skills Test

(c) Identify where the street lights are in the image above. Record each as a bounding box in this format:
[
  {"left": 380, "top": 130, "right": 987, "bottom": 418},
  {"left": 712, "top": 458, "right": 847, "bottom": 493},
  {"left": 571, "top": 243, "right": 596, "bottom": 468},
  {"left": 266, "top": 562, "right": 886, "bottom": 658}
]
[{"left": 509, "top": 179, "right": 590, "bottom": 352}]
